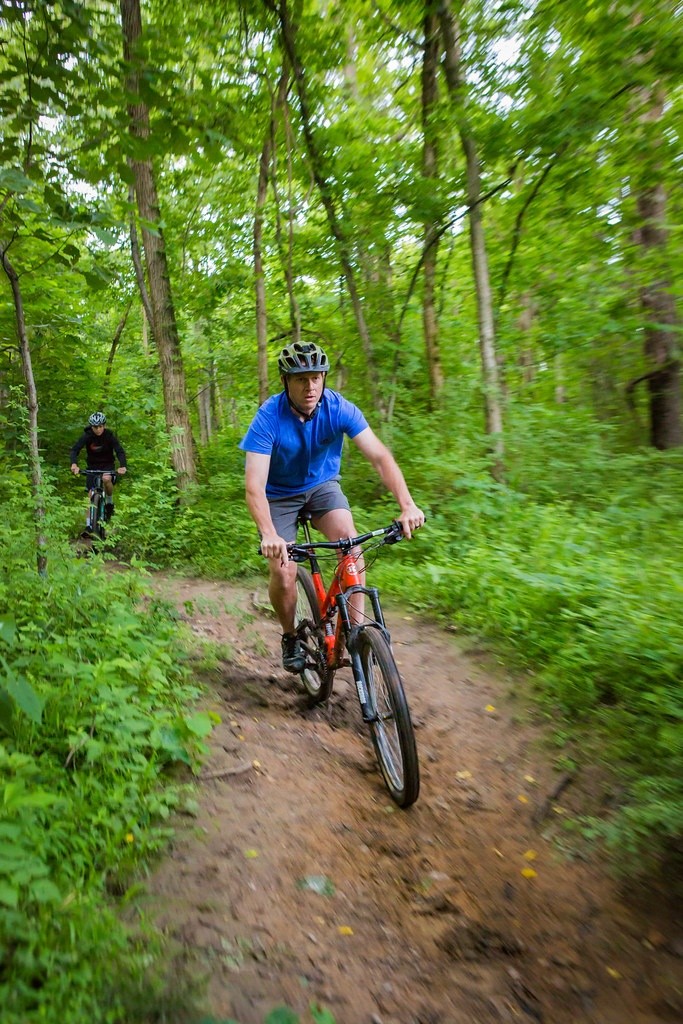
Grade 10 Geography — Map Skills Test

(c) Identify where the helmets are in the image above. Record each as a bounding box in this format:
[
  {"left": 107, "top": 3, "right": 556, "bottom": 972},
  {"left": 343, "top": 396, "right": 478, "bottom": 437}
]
[
  {"left": 89, "top": 412, "right": 107, "bottom": 427},
  {"left": 277, "top": 340, "right": 330, "bottom": 376}
]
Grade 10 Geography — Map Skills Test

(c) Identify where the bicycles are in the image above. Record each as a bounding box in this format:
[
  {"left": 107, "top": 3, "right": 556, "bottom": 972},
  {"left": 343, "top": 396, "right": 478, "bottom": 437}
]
[
  {"left": 72, "top": 467, "right": 120, "bottom": 555},
  {"left": 256, "top": 508, "right": 429, "bottom": 810}
]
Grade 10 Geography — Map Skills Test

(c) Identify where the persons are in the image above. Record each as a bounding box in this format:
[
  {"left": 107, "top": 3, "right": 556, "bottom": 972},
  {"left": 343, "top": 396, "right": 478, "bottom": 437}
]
[
  {"left": 239, "top": 342, "right": 425, "bottom": 674},
  {"left": 71, "top": 412, "right": 127, "bottom": 537}
]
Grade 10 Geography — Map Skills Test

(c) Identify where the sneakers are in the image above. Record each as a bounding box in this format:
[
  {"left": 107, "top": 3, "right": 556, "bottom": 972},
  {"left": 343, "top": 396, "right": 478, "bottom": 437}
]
[
  {"left": 281, "top": 631, "right": 306, "bottom": 673},
  {"left": 106, "top": 502, "right": 114, "bottom": 514},
  {"left": 80, "top": 526, "right": 92, "bottom": 538}
]
